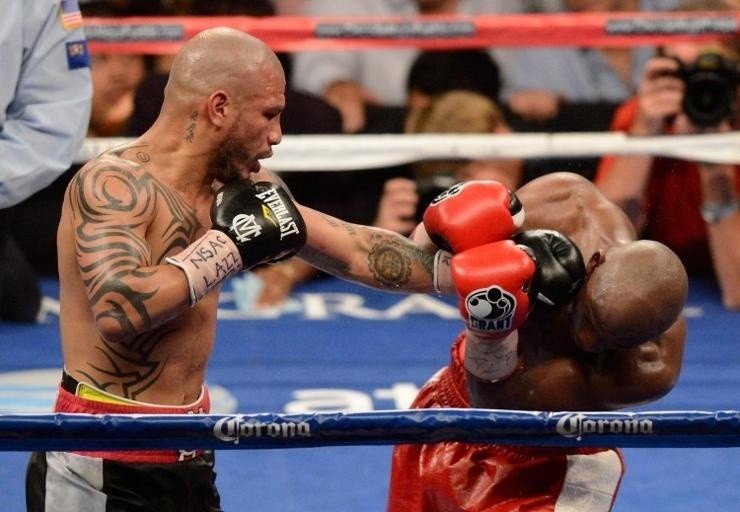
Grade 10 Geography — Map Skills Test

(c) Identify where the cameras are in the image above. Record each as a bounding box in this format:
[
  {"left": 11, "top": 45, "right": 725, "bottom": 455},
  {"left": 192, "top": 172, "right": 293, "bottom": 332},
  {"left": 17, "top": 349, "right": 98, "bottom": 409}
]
[
  {"left": 408, "top": 185, "right": 451, "bottom": 225},
  {"left": 664, "top": 53, "right": 740, "bottom": 127}
]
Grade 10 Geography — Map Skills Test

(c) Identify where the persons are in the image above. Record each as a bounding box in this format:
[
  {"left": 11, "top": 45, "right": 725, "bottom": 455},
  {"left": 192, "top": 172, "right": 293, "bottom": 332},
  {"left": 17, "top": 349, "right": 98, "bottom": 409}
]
[
  {"left": 1, "top": 0, "right": 739, "bottom": 324},
  {"left": 384, "top": 171, "right": 688, "bottom": 512},
  {"left": 25, "top": 28, "right": 590, "bottom": 511}
]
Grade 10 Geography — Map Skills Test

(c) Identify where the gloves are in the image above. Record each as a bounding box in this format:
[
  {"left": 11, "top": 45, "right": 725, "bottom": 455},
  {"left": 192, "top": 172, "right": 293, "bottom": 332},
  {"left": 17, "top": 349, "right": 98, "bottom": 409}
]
[
  {"left": 209, "top": 177, "right": 308, "bottom": 271},
  {"left": 510, "top": 228, "right": 586, "bottom": 311},
  {"left": 422, "top": 178, "right": 525, "bottom": 253},
  {"left": 447, "top": 240, "right": 531, "bottom": 337}
]
